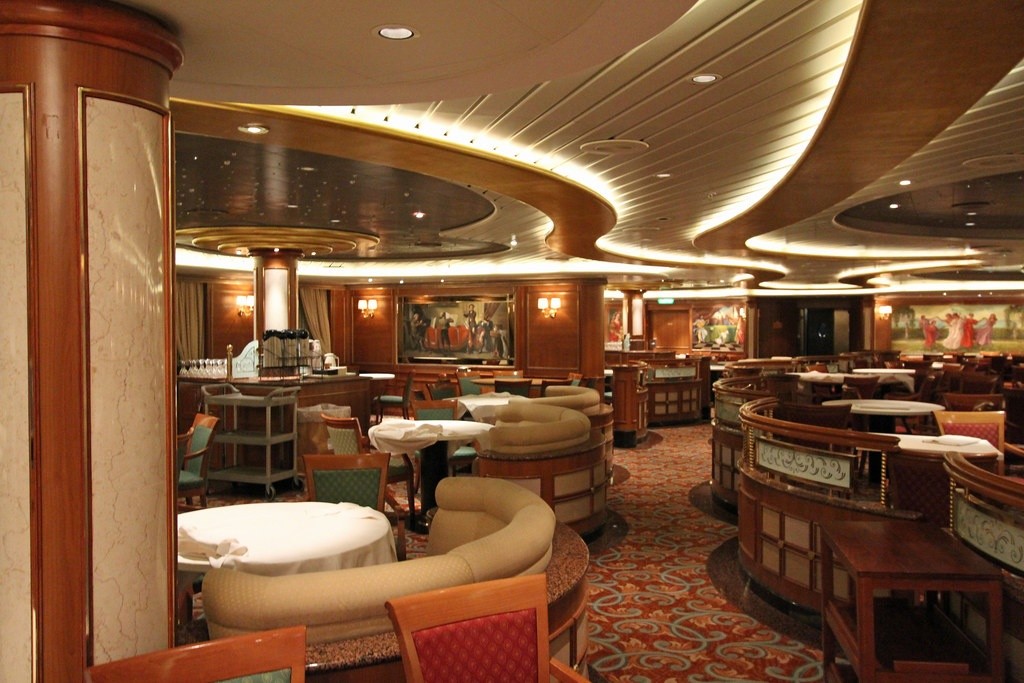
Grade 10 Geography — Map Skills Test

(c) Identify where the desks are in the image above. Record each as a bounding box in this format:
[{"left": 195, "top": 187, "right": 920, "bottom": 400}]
[
  {"left": 861, "top": 433, "right": 1004, "bottom": 490},
  {"left": 852, "top": 368, "right": 915, "bottom": 375},
  {"left": 819, "top": 521, "right": 1005, "bottom": 683},
  {"left": 369, "top": 420, "right": 495, "bottom": 533},
  {"left": 788, "top": 371, "right": 915, "bottom": 398},
  {"left": 345, "top": 373, "right": 396, "bottom": 381},
  {"left": 821, "top": 398, "right": 946, "bottom": 491},
  {"left": 201, "top": 382, "right": 305, "bottom": 500},
  {"left": 442, "top": 392, "right": 527, "bottom": 421},
  {"left": 470, "top": 377, "right": 557, "bottom": 386},
  {"left": 177, "top": 502, "right": 398, "bottom": 624}
]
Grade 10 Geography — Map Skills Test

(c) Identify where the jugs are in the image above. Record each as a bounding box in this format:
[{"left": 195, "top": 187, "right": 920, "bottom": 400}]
[{"left": 324, "top": 353, "right": 340, "bottom": 368}]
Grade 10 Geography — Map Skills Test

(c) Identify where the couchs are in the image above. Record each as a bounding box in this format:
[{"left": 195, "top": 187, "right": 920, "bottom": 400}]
[
  {"left": 508, "top": 385, "right": 614, "bottom": 482},
  {"left": 203, "top": 477, "right": 591, "bottom": 683},
  {"left": 472, "top": 405, "right": 609, "bottom": 533}
]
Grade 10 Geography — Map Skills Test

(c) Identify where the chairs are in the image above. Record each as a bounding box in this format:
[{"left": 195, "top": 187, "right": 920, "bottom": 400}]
[
  {"left": 320, "top": 414, "right": 415, "bottom": 525},
  {"left": 372, "top": 370, "right": 606, "bottom": 424},
  {"left": 176, "top": 413, "right": 221, "bottom": 509},
  {"left": 83, "top": 624, "right": 308, "bottom": 683},
  {"left": 411, "top": 400, "right": 478, "bottom": 493},
  {"left": 385, "top": 573, "right": 592, "bottom": 683},
  {"left": 302, "top": 453, "right": 406, "bottom": 563}
]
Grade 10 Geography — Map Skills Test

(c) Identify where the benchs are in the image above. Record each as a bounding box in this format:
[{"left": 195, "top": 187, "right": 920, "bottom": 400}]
[
  {"left": 710, "top": 350, "right": 1024, "bottom": 682},
  {"left": 604, "top": 349, "right": 746, "bottom": 449}
]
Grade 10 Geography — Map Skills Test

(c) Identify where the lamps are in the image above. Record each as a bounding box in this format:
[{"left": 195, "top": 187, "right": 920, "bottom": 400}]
[
  {"left": 879, "top": 305, "right": 893, "bottom": 320},
  {"left": 236, "top": 294, "right": 254, "bottom": 317},
  {"left": 357, "top": 299, "right": 378, "bottom": 319},
  {"left": 538, "top": 298, "right": 561, "bottom": 319}
]
